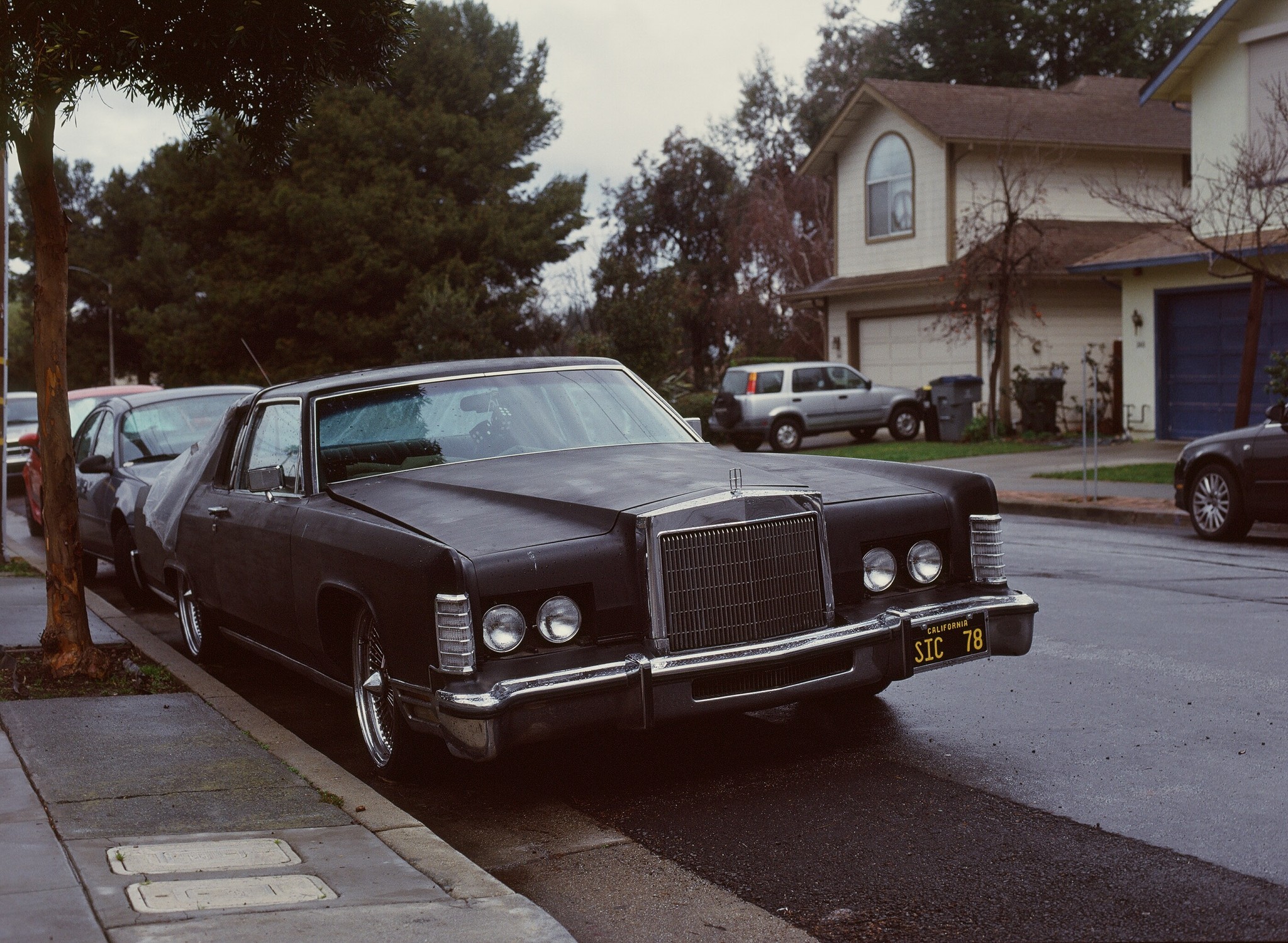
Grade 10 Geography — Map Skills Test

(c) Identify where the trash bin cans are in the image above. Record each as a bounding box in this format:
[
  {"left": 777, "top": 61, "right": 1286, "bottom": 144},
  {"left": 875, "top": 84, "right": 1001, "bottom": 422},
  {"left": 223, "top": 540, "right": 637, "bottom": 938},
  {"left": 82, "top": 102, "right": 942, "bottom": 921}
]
[
  {"left": 1011, "top": 376, "right": 1067, "bottom": 435},
  {"left": 927, "top": 374, "right": 983, "bottom": 441}
]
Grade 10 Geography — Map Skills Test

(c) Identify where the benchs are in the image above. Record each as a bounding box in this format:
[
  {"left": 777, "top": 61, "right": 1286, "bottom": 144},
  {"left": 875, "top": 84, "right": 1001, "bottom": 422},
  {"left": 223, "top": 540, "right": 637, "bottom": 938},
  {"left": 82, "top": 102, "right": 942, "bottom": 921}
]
[{"left": 319, "top": 436, "right": 478, "bottom": 483}]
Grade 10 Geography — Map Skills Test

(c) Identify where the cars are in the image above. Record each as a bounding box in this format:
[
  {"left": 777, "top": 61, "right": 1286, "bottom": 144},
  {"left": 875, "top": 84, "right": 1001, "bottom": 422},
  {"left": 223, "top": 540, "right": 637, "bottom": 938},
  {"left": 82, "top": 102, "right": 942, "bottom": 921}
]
[
  {"left": 1174, "top": 400, "right": 1288, "bottom": 546},
  {"left": 73, "top": 380, "right": 265, "bottom": 609},
  {"left": 7, "top": 390, "right": 40, "bottom": 495},
  {"left": 19, "top": 384, "right": 215, "bottom": 539}
]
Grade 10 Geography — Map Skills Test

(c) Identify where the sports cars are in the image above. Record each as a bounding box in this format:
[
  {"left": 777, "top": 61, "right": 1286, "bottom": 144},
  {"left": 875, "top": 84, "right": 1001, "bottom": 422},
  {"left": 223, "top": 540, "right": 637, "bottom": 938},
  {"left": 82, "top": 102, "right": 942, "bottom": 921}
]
[{"left": 130, "top": 356, "right": 1039, "bottom": 791}]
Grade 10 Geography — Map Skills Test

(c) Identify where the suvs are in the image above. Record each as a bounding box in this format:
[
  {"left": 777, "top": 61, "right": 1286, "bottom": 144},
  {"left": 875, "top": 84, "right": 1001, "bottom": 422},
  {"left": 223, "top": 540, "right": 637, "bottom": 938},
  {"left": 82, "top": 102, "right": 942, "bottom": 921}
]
[{"left": 712, "top": 362, "right": 924, "bottom": 453}]
